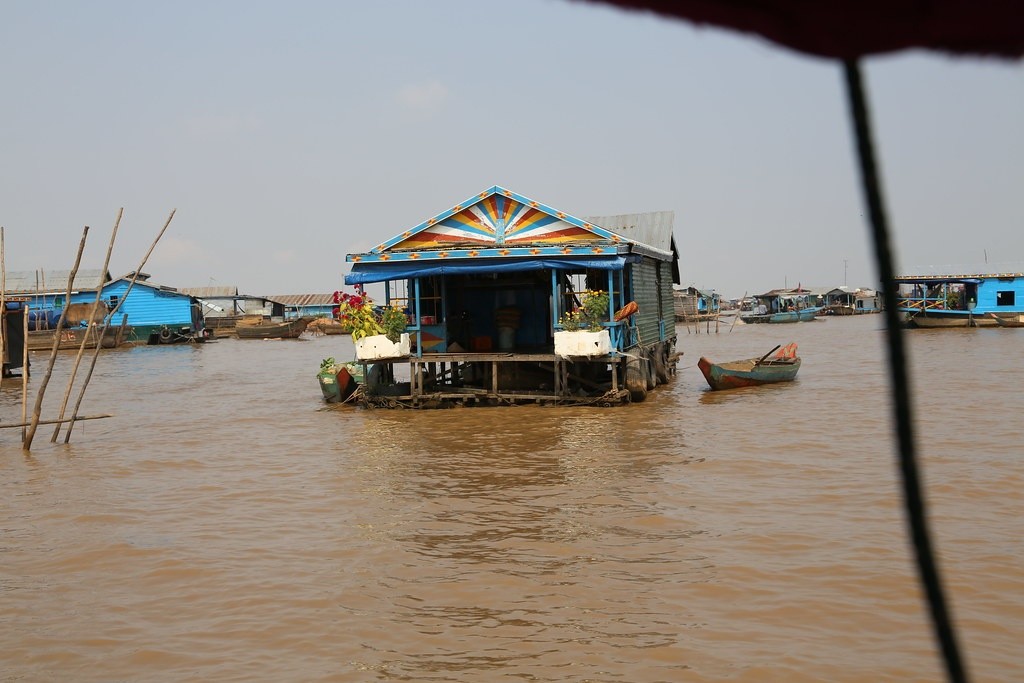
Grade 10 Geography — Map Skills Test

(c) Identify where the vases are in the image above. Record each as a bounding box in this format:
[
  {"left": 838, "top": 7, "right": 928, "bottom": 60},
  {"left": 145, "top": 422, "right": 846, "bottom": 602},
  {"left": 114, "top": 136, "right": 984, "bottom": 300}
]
[
  {"left": 354, "top": 332, "right": 411, "bottom": 361},
  {"left": 554, "top": 329, "right": 609, "bottom": 356}
]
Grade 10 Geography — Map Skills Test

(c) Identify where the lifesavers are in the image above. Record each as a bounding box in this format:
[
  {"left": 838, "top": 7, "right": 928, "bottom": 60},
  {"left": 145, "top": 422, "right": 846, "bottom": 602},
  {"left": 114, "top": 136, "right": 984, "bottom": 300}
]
[
  {"left": 202, "top": 328, "right": 211, "bottom": 338},
  {"left": 159, "top": 328, "right": 173, "bottom": 343},
  {"left": 625, "top": 340, "right": 678, "bottom": 403}
]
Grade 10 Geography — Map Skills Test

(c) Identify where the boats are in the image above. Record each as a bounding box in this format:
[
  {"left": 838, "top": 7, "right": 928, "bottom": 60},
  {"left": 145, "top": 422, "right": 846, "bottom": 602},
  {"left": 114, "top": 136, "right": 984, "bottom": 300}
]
[
  {"left": 234, "top": 315, "right": 316, "bottom": 340},
  {"left": 697, "top": 344, "right": 802, "bottom": 392},
  {"left": 740, "top": 292, "right": 825, "bottom": 325},
  {"left": 822, "top": 286, "right": 886, "bottom": 316},
  {"left": 315, "top": 323, "right": 355, "bottom": 336},
  {"left": 893, "top": 273, "right": 1024, "bottom": 331},
  {"left": 25, "top": 322, "right": 132, "bottom": 350}
]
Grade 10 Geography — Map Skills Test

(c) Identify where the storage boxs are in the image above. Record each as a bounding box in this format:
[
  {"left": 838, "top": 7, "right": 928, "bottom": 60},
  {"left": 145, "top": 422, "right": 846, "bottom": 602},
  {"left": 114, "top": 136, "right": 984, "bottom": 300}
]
[{"left": 468, "top": 334, "right": 493, "bottom": 352}]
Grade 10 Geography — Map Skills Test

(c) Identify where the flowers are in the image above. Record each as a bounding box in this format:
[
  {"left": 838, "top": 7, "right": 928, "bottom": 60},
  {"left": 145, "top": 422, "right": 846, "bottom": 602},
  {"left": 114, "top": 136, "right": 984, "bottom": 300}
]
[
  {"left": 557, "top": 288, "right": 612, "bottom": 330},
  {"left": 330, "top": 283, "right": 409, "bottom": 331}
]
